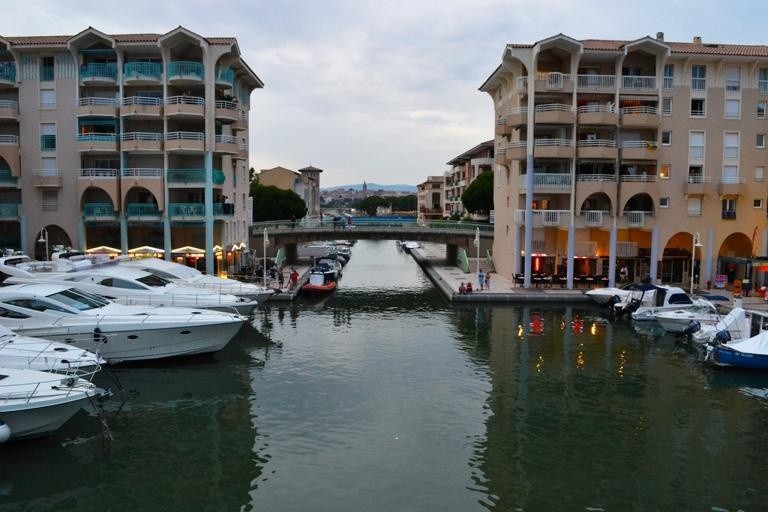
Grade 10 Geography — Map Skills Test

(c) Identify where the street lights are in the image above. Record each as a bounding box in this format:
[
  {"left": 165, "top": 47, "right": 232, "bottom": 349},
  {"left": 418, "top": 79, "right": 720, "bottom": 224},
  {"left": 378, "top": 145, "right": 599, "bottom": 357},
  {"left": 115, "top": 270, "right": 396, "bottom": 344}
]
[
  {"left": 260, "top": 225, "right": 270, "bottom": 289},
  {"left": 689, "top": 229, "right": 703, "bottom": 294},
  {"left": 36, "top": 225, "right": 50, "bottom": 260},
  {"left": 472, "top": 225, "right": 481, "bottom": 292}
]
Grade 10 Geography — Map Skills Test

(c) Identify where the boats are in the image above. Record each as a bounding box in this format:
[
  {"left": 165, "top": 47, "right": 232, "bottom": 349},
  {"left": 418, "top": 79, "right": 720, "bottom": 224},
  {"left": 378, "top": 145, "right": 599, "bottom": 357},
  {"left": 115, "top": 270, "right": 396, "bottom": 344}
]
[
  {"left": 298, "top": 238, "right": 356, "bottom": 294},
  {"left": 396, "top": 240, "right": 420, "bottom": 253},
  {"left": 582, "top": 280, "right": 767, "bottom": 371}
]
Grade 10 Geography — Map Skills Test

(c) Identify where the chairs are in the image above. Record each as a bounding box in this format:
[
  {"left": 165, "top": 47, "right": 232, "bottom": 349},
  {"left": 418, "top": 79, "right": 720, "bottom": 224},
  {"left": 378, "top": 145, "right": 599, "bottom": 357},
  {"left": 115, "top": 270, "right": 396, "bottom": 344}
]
[
  {"left": 231, "top": 262, "right": 263, "bottom": 284},
  {"left": 511, "top": 271, "right": 612, "bottom": 291}
]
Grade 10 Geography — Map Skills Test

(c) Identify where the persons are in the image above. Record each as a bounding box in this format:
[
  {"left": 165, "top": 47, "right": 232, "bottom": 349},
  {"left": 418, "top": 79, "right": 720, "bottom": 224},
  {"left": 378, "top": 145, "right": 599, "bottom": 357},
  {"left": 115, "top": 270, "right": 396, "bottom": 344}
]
[
  {"left": 342, "top": 218, "right": 345, "bottom": 229},
  {"left": 457, "top": 282, "right": 465, "bottom": 294},
  {"left": 464, "top": 282, "right": 473, "bottom": 294},
  {"left": 332, "top": 216, "right": 337, "bottom": 229},
  {"left": 477, "top": 269, "right": 484, "bottom": 291},
  {"left": 277, "top": 266, "right": 284, "bottom": 288},
  {"left": 291, "top": 214, "right": 295, "bottom": 228},
  {"left": 484, "top": 270, "right": 490, "bottom": 290},
  {"left": 290, "top": 269, "right": 299, "bottom": 288},
  {"left": 347, "top": 216, "right": 352, "bottom": 230}
]
